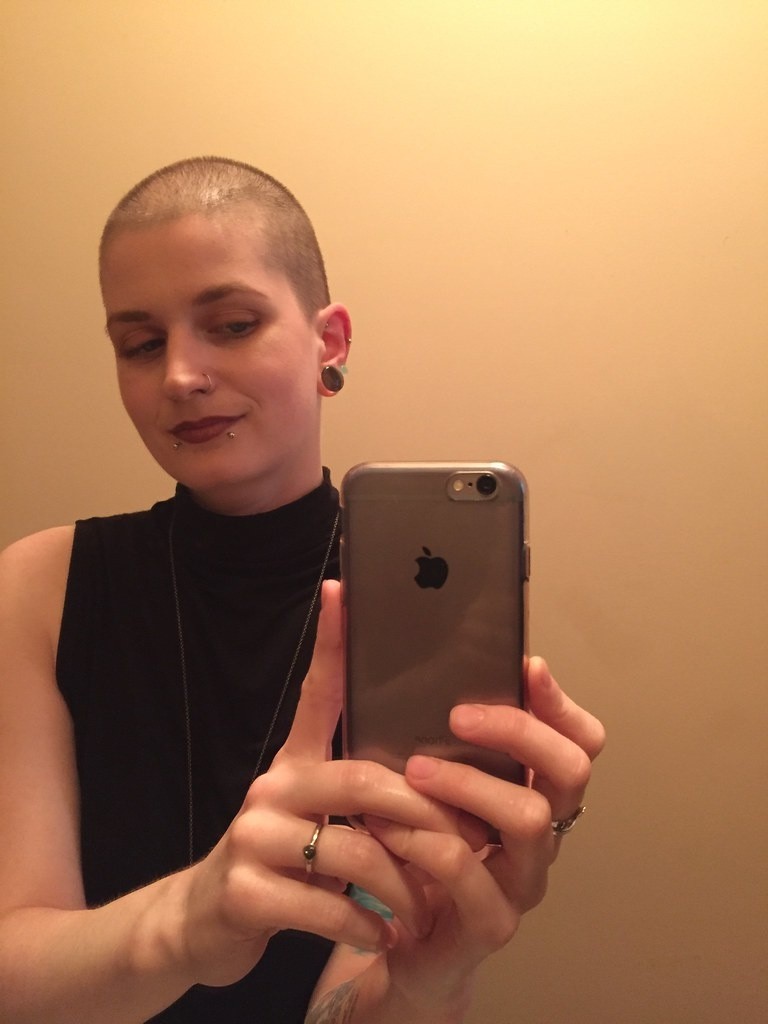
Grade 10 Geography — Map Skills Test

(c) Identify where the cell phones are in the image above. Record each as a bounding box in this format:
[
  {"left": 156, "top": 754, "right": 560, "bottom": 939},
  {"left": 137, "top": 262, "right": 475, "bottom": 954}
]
[{"left": 337, "top": 460, "right": 530, "bottom": 845}]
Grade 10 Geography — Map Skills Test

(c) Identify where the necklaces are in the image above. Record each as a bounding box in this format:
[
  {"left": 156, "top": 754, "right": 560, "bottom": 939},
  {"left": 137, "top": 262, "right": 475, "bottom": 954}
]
[{"left": 159, "top": 492, "right": 344, "bottom": 868}]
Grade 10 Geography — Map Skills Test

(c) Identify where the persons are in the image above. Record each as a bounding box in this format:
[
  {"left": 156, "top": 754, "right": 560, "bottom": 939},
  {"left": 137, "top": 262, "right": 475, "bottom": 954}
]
[{"left": 0, "top": 156, "right": 608, "bottom": 1024}]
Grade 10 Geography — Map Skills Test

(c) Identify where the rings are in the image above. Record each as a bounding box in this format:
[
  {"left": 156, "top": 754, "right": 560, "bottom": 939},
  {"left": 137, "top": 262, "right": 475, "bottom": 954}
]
[
  {"left": 304, "top": 823, "right": 323, "bottom": 873},
  {"left": 552, "top": 807, "right": 586, "bottom": 837}
]
